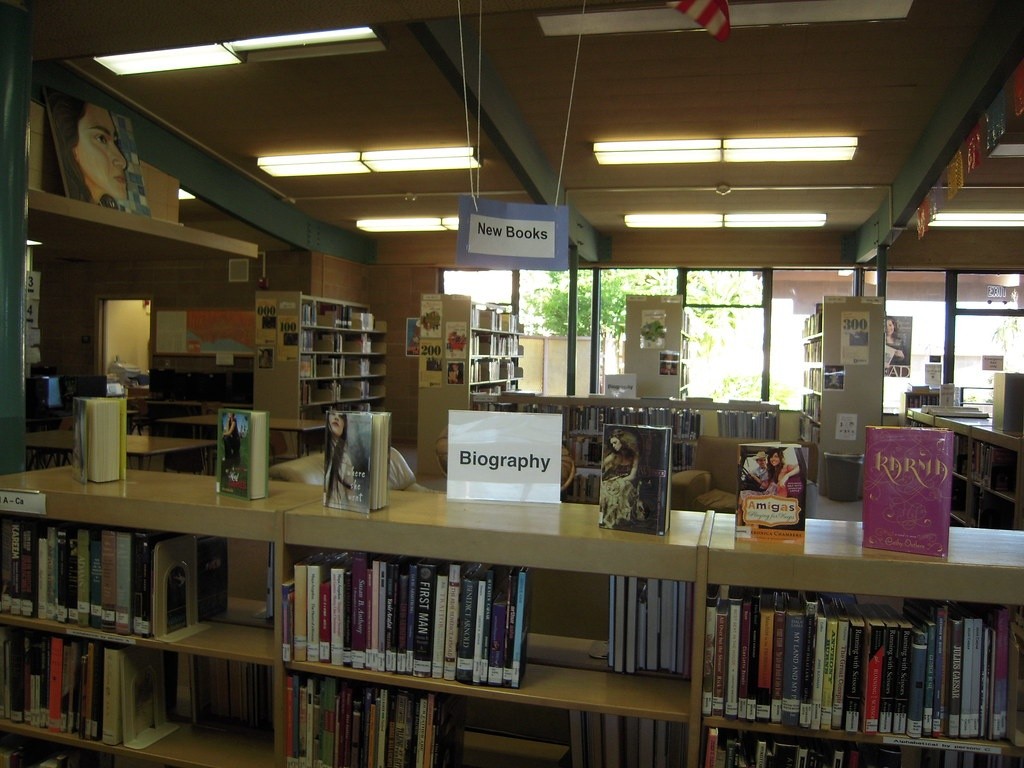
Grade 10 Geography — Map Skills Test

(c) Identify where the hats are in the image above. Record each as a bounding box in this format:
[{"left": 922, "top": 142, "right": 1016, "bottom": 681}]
[{"left": 752, "top": 451, "right": 768, "bottom": 461}]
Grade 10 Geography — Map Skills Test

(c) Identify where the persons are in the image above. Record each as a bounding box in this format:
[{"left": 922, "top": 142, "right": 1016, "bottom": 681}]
[
  {"left": 223, "top": 411, "right": 241, "bottom": 466},
  {"left": 750, "top": 449, "right": 799, "bottom": 497},
  {"left": 448, "top": 363, "right": 463, "bottom": 384},
  {"left": 660, "top": 355, "right": 677, "bottom": 375},
  {"left": 324, "top": 412, "right": 357, "bottom": 510},
  {"left": 885, "top": 317, "right": 906, "bottom": 365},
  {"left": 600, "top": 429, "right": 640, "bottom": 529}
]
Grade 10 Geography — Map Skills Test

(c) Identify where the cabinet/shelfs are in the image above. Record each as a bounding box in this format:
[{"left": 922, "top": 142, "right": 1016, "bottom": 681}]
[
  {"left": 0, "top": 463, "right": 1024, "bottom": 768},
  {"left": 798, "top": 295, "right": 885, "bottom": 484},
  {"left": 418, "top": 293, "right": 523, "bottom": 476},
  {"left": 908, "top": 407, "right": 1024, "bottom": 532},
  {"left": 626, "top": 293, "right": 690, "bottom": 401},
  {"left": 256, "top": 289, "right": 387, "bottom": 443},
  {"left": 472, "top": 392, "right": 779, "bottom": 502}
]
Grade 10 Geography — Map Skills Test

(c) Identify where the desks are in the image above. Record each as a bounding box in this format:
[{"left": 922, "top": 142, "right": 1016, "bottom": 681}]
[
  {"left": 24, "top": 430, "right": 218, "bottom": 474},
  {"left": 158, "top": 415, "right": 327, "bottom": 457}
]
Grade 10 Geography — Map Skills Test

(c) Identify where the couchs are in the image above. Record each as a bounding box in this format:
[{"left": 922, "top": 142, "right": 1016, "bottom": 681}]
[
  {"left": 269, "top": 443, "right": 445, "bottom": 494},
  {"left": 672, "top": 435, "right": 818, "bottom": 520}
]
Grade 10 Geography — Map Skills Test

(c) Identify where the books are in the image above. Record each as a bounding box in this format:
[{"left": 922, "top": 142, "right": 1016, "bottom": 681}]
[
  {"left": 473, "top": 401, "right": 701, "bottom": 502},
  {"left": 216, "top": 408, "right": 269, "bottom": 500},
  {"left": 681, "top": 312, "right": 690, "bottom": 399},
  {"left": 861, "top": 425, "right": 955, "bottom": 558},
  {"left": 323, "top": 410, "right": 391, "bottom": 514},
  {"left": 470, "top": 304, "right": 519, "bottom": 394},
  {"left": 906, "top": 393, "right": 1017, "bottom": 530},
  {"left": 598, "top": 422, "right": 672, "bottom": 536},
  {"left": 72, "top": 398, "right": 127, "bottom": 484},
  {"left": 798, "top": 311, "right": 823, "bottom": 443},
  {"left": 716, "top": 409, "right": 775, "bottom": 439},
  {"left": 0, "top": 514, "right": 1024, "bottom": 768},
  {"left": 734, "top": 441, "right": 808, "bottom": 542},
  {"left": 299, "top": 299, "right": 374, "bottom": 420}
]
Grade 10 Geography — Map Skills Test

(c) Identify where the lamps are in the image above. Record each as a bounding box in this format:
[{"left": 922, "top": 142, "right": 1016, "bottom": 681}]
[
  {"left": 356, "top": 215, "right": 462, "bottom": 233},
  {"left": 590, "top": 139, "right": 858, "bottom": 164},
  {"left": 537, "top": 0, "right": 914, "bottom": 39},
  {"left": 927, "top": 209, "right": 1024, "bottom": 228},
  {"left": 254, "top": 147, "right": 483, "bottom": 179},
  {"left": 91, "top": 22, "right": 391, "bottom": 77},
  {"left": 623, "top": 211, "right": 827, "bottom": 229}
]
